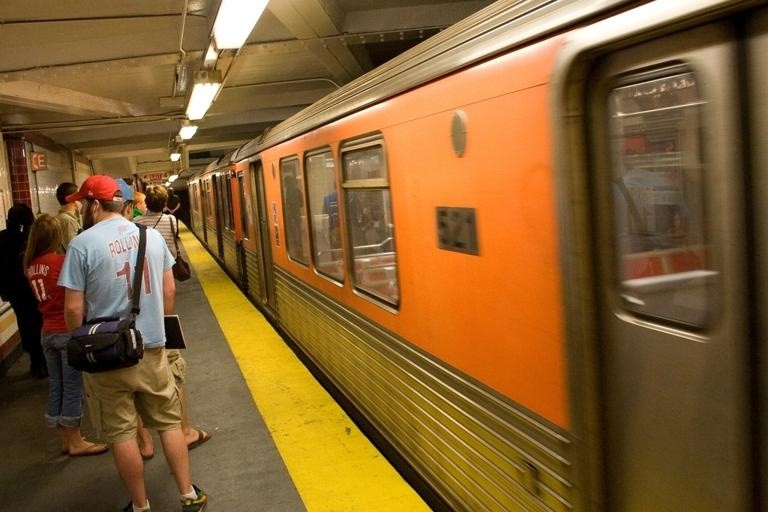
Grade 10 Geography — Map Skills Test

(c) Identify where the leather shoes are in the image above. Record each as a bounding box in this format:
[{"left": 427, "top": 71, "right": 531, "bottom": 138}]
[{"left": 35, "top": 368, "right": 48, "bottom": 379}]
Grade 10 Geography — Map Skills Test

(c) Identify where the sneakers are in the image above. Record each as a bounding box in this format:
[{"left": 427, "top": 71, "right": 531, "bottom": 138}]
[
  {"left": 124, "top": 500, "right": 152, "bottom": 512},
  {"left": 180, "top": 486, "right": 207, "bottom": 512}
]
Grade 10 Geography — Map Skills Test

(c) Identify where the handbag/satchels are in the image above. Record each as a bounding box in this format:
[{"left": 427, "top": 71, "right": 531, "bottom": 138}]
[
  {"left": 172, "top": 256, "right": 190, "bottom": 282},
  {"left": 66, "top": 315, "right": 144, "bottom": 373}
]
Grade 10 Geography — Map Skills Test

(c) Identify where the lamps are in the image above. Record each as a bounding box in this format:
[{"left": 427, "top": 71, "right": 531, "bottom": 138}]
[
  {"left": 185, "top": 71, "right": 223, "bottom": 121},
  {"left": 209, "top": 0, "right": 268, "bottom": 54},
  {"left": 178, "top": 120, "right": 199, "bottom": 142}
]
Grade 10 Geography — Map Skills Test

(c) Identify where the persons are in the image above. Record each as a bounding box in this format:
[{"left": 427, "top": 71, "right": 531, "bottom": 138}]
[
  {"left": 282, "top": 175, "right": 305, "bottom": 261},
  {"left": 55, "top": 182, "right": 85, "bottom": 256},
  {"left": 57, "top": 176, "right": 207, "bottom": 512},
  {"left": 122, "top": 178, "right": 180, "bottom": 261},
  {"left": 24, "top": 214, "right": 108, "bottom": 457},
  {"left": 322, "top": 176, "right": 370, "bottom": 258},
  {"left": 1, "top": 203, "right": 51, "bottom": 380},
  {"left": 615, "top": 133, "right": 686, "bottom": 254},
  {"left": 116, "top": 178, "right": 210, "bottom": 460}
]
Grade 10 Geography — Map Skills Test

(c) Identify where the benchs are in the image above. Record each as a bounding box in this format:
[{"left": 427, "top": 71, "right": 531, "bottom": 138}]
[
  {"left": 317, "top": 244, "right": 400, "bottom": 305},
  {"left": 621, "top": 243, "right": 710, "bottom": 315}
]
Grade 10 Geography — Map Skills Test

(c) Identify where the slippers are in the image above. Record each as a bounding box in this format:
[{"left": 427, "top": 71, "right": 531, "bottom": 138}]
[
  {"left": 138, "top": 443, "right": 153, "bottom": 460},
  {"left": 187, "top": 430, "right": 210, "bottom": 450},
  {"left": 69, "top": 443, "right": 108, "bottom": 457}
]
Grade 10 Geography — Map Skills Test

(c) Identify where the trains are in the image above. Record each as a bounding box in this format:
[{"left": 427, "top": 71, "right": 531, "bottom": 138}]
[{"left": 183, "top": 1, "right": 767, "bottom": 512}]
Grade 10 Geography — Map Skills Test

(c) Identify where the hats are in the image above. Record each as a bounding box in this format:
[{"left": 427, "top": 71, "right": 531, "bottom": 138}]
[
  {"left": 115, "top": 178, "right": 134, "bottom": 203},
  {"left": 65, "top": 175, "right": 122, "bottom": 203}
]
